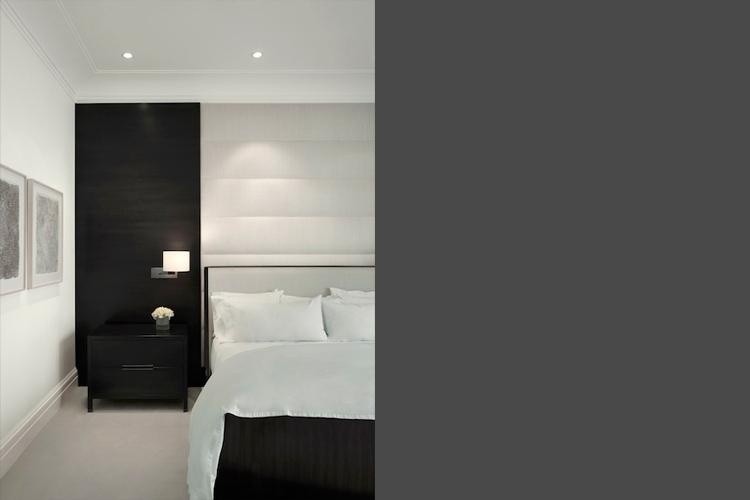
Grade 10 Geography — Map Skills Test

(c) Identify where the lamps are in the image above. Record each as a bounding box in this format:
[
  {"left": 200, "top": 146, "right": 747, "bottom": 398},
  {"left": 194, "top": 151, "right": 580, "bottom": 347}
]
[{"left": 151, "top": 250, "right": 191, "bottom": 279}]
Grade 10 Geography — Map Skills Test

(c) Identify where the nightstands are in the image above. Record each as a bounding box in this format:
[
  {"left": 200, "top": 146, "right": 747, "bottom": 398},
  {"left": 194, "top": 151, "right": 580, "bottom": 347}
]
[{"left": 87, "top": 318, "right": 188, "bottom": 413}]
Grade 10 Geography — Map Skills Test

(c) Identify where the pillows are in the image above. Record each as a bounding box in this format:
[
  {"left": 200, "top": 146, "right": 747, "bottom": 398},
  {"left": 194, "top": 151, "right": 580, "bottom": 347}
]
[{"left": 211, "top": 287, "right": 375, "bottom": 342}]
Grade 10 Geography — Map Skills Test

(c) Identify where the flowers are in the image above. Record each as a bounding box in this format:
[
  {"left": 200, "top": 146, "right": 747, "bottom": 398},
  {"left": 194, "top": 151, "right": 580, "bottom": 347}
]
[{"left": 151, "top": 306, "right": 174, "bottom": 319}]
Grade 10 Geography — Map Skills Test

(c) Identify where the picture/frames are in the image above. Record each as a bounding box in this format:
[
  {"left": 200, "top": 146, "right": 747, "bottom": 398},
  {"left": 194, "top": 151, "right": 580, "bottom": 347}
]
[
  {"left": 0, "top": 164, "right": 26, "bottom": 296},
  {"left": 27, "top": 178, "right": 64, "bottom": 288}
]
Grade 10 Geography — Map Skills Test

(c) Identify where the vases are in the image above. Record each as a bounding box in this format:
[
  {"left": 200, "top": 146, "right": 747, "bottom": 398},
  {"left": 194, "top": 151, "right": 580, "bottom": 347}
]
[{"left": 156, "top": 320, "right": 170, "bottom": 330}]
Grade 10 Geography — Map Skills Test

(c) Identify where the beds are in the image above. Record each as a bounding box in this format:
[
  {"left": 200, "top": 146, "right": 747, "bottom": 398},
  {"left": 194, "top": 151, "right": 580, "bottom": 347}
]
[{"left": 185, "top": 265, "right": 376, "bottom": 500}]
